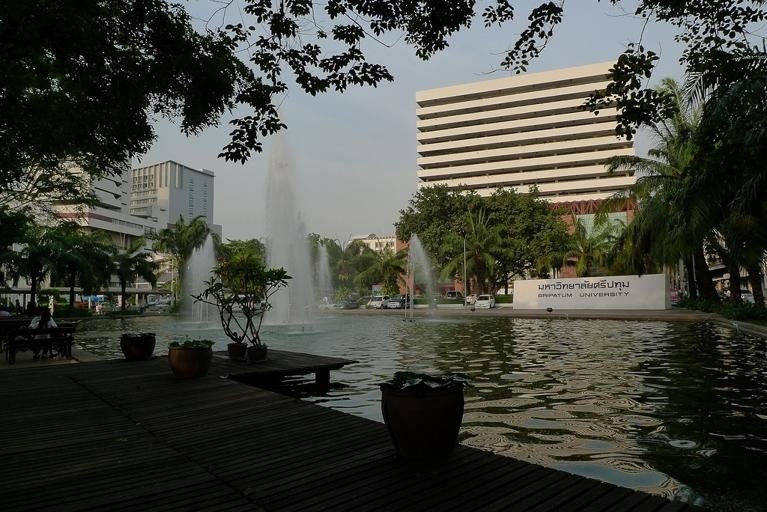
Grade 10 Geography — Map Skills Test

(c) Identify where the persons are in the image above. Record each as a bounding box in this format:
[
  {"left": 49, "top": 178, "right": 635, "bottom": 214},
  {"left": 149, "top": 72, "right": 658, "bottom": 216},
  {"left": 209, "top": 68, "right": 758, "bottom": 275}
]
[
  {"left": 28, "top": 305, "right": 60, "bottom": 331},
  {"left": 0, "top": 304, "right": 12, "bottom": 316},
  {"left": 95, "top": 302, "right": 102, "bottom": 315},
  {"left": 469, "top": 295, "right": 476, "bottom": 311},
  {"left": 26, "top": 309, "right": 53, "bottom": 360}
]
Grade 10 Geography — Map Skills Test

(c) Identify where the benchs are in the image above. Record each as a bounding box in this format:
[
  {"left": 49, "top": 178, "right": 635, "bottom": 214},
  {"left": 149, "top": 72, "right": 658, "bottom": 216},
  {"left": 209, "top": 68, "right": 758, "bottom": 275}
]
[{"left": 0, "top": 315, "right": 75, "bottom": 365}]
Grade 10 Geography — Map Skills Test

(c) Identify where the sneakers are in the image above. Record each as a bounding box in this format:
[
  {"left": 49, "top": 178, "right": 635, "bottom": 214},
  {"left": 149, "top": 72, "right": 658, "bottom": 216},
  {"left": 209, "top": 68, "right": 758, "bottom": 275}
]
[
  {"left": 40, "top": 356, "right": 46, "bottom": 360},
  {"left": 33, "top": 355, "right": 40, "bottom": 359}
]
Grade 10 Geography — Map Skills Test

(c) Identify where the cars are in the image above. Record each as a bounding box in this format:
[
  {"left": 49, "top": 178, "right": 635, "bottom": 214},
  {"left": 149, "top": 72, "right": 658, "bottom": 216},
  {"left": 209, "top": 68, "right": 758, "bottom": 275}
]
[
  {"left": 369, "top": 295, "right": 389, "bottom": 309},
  {"left": 387, "top": 294, "right": 410, "bottom": 309},
  {"left": 467, "top": 293, "right": 495, "bottom": 309},
  {"left": 444, "top": 291, "right": 462, "bottom": 300},
  {"left": 321, "top": 295, "right": 359, "bottom": 310}
]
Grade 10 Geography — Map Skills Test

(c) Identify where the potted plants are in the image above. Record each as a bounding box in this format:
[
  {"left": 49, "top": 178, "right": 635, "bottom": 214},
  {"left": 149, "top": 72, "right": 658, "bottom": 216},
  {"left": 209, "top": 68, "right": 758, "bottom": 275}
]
[
  {"left": 168, "top": 238, "right": 293, "bottom": 382},
  {"left": 380, "top": 370, "right": 464, "bottom": 466},
  {"left": 120, "top": 332, "right": 156, "bottom": 361}
]
